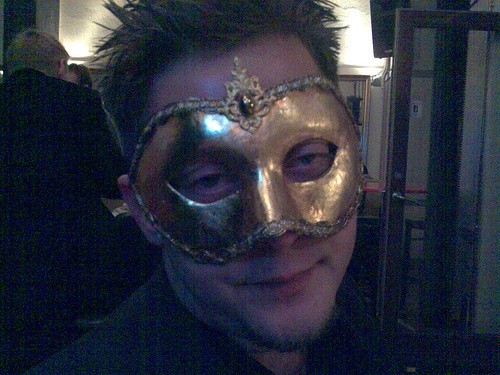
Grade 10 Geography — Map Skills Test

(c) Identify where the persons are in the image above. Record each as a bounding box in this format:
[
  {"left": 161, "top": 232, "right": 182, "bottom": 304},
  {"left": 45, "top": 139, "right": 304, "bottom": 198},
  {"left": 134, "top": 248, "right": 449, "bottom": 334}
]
[
  {"left": 26, "top": 0, "right": 398, "bottom": 375},
  {"left": 0, "top": 29, "right": 123, "bottom": 375}
]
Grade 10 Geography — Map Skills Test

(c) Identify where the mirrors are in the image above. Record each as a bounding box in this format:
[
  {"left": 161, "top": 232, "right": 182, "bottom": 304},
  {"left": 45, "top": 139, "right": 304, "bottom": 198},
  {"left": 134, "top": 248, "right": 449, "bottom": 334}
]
[{"left": 337, "top": 75, "right": 371, "bottom": 167}]
